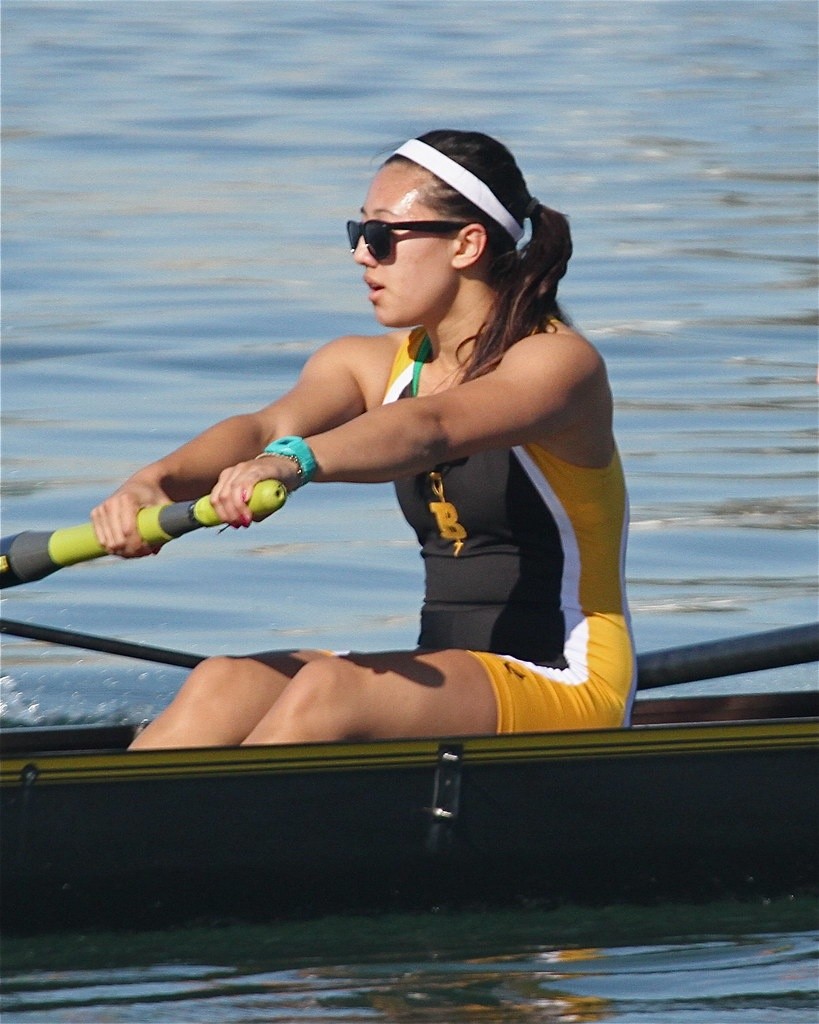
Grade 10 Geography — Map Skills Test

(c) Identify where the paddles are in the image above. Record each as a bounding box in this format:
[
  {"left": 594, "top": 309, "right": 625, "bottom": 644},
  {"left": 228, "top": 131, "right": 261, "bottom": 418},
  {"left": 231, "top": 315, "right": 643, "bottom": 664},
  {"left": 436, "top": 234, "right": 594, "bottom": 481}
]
[{"left": 1, "top": 482, "right": 290, "bottom": 585}]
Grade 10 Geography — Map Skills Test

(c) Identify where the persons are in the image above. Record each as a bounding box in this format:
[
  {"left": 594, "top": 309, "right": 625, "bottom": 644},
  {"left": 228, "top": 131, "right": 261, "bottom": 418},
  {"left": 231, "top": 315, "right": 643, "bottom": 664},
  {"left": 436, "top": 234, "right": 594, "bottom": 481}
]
[{"left": 91, "top": 129, "right": 638, "bottom": 750}]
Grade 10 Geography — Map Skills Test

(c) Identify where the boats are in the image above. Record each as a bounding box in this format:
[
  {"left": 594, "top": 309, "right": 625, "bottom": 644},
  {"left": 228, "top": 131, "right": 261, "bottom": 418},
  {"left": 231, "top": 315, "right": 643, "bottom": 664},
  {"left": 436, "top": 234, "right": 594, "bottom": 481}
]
[{"left": 0, "top": 691, "right": 819, "bottom": 929}]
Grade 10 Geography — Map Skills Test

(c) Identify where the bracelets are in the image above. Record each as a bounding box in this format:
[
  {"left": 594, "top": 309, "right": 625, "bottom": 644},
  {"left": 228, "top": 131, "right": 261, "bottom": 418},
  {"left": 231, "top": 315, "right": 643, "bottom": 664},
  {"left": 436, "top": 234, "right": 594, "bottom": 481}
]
[{"left": 257, "top": 453, "right": 302, "bottom": 484}]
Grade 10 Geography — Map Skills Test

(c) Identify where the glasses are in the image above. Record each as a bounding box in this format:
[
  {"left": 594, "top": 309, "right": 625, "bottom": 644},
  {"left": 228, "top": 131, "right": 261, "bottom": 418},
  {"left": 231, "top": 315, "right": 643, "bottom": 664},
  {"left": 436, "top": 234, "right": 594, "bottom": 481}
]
[{"left": 346, "top": 218, "right": 492, "bottom": 261}]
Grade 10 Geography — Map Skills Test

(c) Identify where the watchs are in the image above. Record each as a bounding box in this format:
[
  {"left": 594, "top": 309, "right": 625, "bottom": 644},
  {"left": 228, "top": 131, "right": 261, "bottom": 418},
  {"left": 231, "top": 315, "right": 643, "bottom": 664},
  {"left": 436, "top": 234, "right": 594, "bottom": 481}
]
[{"left": 266, "top": 435, "right": 317, "bottom": 484}]
